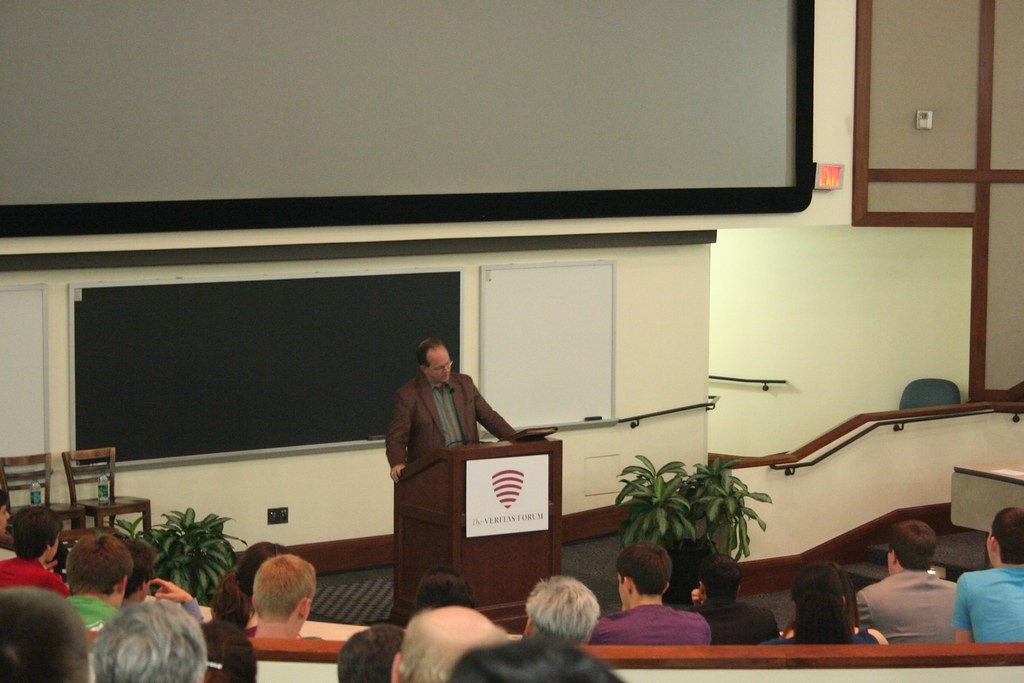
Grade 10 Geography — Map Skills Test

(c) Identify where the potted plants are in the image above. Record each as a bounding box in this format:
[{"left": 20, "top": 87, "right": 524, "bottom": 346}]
[{"left": 614, "top": 454, "right": 774, "bottom": 598}]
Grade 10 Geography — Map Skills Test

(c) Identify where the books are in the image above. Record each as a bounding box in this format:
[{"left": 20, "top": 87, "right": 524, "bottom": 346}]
[{"left": 497, "top": 427, "right": 557, "bottom": 439}]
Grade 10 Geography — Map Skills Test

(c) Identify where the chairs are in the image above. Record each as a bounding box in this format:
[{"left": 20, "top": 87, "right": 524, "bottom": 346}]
[
  {"left": 0, "top": 453, "right": 86, "bottom": 532},
  {"left": 900, "top": 379, "right": 960, "bottom": 409},
  {"left": 62, "top": 447, "right": 151, "bottom": 533}
]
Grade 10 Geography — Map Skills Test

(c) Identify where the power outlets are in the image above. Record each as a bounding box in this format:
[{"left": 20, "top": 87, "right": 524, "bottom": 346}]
[{"left": 268, "top": 507, "right": 289, "bottom": 525}]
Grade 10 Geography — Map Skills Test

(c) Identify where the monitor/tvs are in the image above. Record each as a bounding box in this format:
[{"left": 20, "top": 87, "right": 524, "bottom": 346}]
[{"left": 499, "top": 427, "right": 558, "bottom": 442}]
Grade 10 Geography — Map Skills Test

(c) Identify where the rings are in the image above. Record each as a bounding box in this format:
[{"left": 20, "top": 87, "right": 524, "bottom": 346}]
[{"left": 391, "top": 472, "right": 394, "bottom": 475}]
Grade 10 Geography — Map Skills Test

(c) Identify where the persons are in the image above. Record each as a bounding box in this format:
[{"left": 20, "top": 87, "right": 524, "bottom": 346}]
[
  {"left": 758, "top": 562, "right": 888, "bottom": 645},
  {"left": 951, "top": 507, "right": 1024, "bottom": 642},
  {"left": 211, "top": 541, "right": 323, "bottom": 639},
  {"left": 854, "top": 520, "right": 957, "bottom": 644},
  {"left": 0, "top": 505, "right": 70, "bottom": 597},
  {"left": 0, "top": 586, "right": 89, "bottom": 683},
  {"left": 686, "top": 553, "right": 780, "bottom": 645},
  {"left": 93, "top": 600, "right": 207, "bottom": 683},
  {"left": 120, "top": 537, "right": 204, "bottom": 624},
  {"left": 65, "top": 532, "right": 133, "bottom": 683},
  {"left": 447, "top": 639, "right": 624, "bottom": 683},
  {"left": 391, "top": 606, "right": 508, "bottom": 683},
  {"left": 0, "top": 489, "right": 17, "bottom": 560},
  {"left": 386, "top": 339, "right": 516, "bottom": 483},
  {"left": 589, "top": 542, "right": 712, "bottom": 645},
  {"left": 524, "top": 576, "right": 600, "bottom": 644},
  {"left": 337, "top": 624, "right": 405, "bottom": 683},
  {"left": 198, "top": 619, "right": 257, "bottom": 683}
]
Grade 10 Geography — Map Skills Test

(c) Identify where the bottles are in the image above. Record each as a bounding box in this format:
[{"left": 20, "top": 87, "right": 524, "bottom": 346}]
[
  {"left": 28, "top": 476, "right": 43, "bottom": 506},
  {"left": 98, "top": 469, "right": 110, "bottom": 505}
]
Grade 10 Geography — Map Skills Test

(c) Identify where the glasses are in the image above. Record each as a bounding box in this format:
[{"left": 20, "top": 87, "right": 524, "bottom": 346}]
[{"left": 425, "top": 360, "right": 453, "bottom": 371}]
